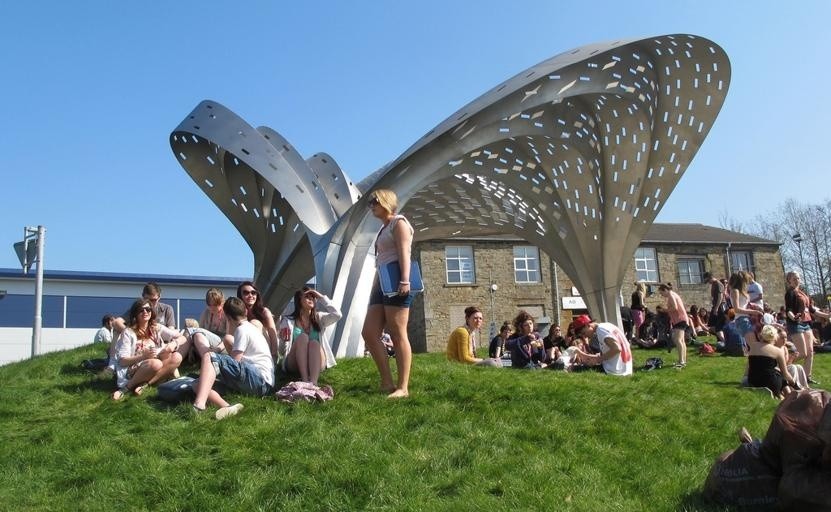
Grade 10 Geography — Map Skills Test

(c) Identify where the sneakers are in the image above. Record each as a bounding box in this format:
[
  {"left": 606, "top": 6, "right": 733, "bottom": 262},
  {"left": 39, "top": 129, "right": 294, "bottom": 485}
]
[
  {"left": 215, "top": 403, "right": 244, "bottom": 420},
  {"left": 807, "top": 379, "right": 817, "bottom": 384},
  {"left": 135, "top": 382, "right": 148, "bottom": 396},
  {"left": 113, "top": 389, "right": 125, "bottom": 400}
]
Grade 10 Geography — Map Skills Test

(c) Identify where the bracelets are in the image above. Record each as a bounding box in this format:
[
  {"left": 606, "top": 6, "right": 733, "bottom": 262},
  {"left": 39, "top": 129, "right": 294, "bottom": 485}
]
[{"left": 399, "top": 281, "right": 411, "bottom": 285}]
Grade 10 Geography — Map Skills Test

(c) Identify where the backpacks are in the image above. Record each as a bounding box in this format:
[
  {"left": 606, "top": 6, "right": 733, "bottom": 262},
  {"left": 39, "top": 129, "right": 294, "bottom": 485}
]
[{"left": 275, "top": 381, "right": 334, "bottom": 406}]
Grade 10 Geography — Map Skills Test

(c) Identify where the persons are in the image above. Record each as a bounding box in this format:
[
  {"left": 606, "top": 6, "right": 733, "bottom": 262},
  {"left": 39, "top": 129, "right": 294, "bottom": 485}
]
[
  {"left": 274, "top": 286, "right": 342, "bottom": 389},
  {"left": 234, "top": 281, "right": 279, "bottom": 357},
  {"left": 94, "top": 315, "right": 113, "bottom": 344},
  {"left": 96, "top": 282, "right": 180, "bottom": 379},
  {"left": 111, "top": 300, "right": 183, "bottom": 401},
  {"left": 631, "top": 271, "right": 831, "bottom": 398},
  {"left": 178, "top": 285, "right": 230, "bottom": 361},
  {"left": 379, "top": 328, "right": 394, "bottom": 358},
  {"left": 448, "top": 306, "right": 632, "bottom": 376},
  {"left": 361, "top": 188, "right": 416, "bottom": 402},
  {"left": 189, "top": 296, "right": 275, "bottom": 421}
]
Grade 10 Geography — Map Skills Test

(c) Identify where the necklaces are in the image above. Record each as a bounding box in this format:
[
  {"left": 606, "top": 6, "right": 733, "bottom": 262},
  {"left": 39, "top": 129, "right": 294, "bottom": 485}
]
[{"left": 137, "top": 325, "right": 149, "bottom": 338}]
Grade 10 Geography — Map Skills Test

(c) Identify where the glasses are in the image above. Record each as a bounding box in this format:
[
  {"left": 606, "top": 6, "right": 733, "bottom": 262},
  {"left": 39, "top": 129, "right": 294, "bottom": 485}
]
[
  {"left": 150, "top": 296, "right": 160, "bottom": 302},
  {"left": 243, "top": 291, "right": 257, "bottom": 295},
  {"left": 136, "top": 306, "right": 152, "bottom": 313},
  {"left": 368, "top": 198, "right": 378, "bottom": 208}
]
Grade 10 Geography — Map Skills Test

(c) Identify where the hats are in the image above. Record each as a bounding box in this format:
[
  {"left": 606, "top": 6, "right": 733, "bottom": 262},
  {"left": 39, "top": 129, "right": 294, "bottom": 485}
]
[
  {"left": 573, "top": 314, "right": 592, "bottom": 335},
  {"left": 703, "top": 271, "right": 711, "bottom": 284}
]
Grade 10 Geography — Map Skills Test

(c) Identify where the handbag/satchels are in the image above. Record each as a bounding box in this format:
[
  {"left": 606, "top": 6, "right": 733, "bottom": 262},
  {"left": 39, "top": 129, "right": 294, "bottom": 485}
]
[{"left": 641, "top": 357, "right": 663, "bottom": 371}]
